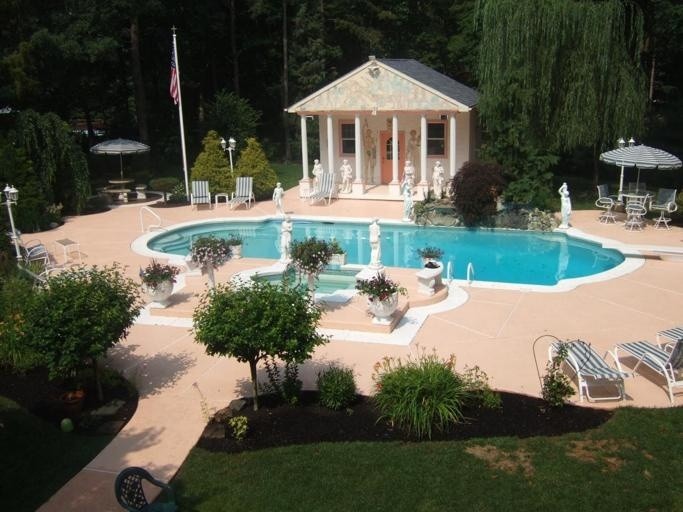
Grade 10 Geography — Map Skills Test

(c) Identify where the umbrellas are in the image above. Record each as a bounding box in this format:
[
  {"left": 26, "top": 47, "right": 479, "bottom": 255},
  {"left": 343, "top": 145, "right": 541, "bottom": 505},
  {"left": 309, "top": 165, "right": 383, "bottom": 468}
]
[
  {"left": 90, "top": 138, "right": 151, "bottom": 178},
  {"left": 600, "top": 143, "right": 682, "bottom": 192}
]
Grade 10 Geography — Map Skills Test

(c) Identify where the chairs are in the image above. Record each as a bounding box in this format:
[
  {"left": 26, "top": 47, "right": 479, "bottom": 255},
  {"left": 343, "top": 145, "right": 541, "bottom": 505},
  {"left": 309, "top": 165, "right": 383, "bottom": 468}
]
[
  {"left": 227, "top": 177, "right": 255, "bottom": 210},
  {"left": 308, "top": 173, "right": 338, "bottom": 206},
  {"left": 190, "top": 180, "right": 210, "bottom": 210},
  {"left": 547, "top": 326, "right": 682, "bottom": 402},
  {"left": 595, "top": 182, "right": 677, "bottom": 231},
  {"left": 237, "top": 225, "right": 256, "bottom": 238},
  {"left": 114, "top": 465, "right": 177, "bottom": 511},
  {"left": 6, "top": 230, "right": 52, "bottom": 280}
]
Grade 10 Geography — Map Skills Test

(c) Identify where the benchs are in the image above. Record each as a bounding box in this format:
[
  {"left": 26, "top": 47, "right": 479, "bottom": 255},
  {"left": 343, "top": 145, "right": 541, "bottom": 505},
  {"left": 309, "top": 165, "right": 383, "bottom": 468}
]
[{"left": 94, "top": 178, "right": 147, "bottom": 202}]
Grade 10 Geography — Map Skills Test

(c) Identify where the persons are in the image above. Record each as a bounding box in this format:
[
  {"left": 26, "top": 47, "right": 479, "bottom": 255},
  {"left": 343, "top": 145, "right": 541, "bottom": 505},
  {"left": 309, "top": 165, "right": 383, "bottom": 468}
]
[
  {"left": 339, "top": 159, "right": 352, "bottom": 190},
  {"left": 273, "top": 182, "right": 284, "bottom": 215},
  {"left": 559, "top": 183, "right": 571, "bottom": 225},
  {"left": 280, "top": 217, "right": 293, "bottom": 259},
  {"left": 402, "top": 129, "right": 445, "bottom": 218},
  {"left": 368, "top": 218, "right": 381, "bottom": 267},
  {"left": 313, "top": 159, "right": 322, "bottom": 179},
  {"left": 362, "top": 122, "right": 375, "bottom": 185}
]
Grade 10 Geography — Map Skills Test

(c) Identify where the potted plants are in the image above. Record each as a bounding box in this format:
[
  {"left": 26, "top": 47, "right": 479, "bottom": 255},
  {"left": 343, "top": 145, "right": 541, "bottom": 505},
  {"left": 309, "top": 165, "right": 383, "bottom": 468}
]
[
  {"left": 227, "top": 239, "right": 241, "bottom": 259},
  {"left": 322, "top": 239, "right": 346, "bottom": 265},
  {"left": 416, "top": 245, "right": 442, "bottom": 265}
]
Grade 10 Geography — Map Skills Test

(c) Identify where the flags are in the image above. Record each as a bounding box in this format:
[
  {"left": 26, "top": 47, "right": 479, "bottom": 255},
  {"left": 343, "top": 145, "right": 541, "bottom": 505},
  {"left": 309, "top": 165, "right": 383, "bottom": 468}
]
[{"left": 169, "top": 39, "right": 178, "bottom": 103}]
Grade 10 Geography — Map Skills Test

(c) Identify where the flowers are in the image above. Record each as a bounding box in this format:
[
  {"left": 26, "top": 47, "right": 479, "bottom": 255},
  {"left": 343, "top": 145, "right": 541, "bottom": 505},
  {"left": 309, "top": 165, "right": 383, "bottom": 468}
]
[
  {"left": 353, "top": 270, "right": 407, "bottom": 303},
  {"left": 138, "top": 259, "right": 181, "bottom": 288}
]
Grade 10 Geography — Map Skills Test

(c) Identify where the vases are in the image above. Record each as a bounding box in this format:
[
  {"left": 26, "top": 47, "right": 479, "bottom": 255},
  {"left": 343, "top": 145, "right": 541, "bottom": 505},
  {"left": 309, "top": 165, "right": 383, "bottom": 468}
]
[
  {"left": 141, "top": 279, "right": 172, "bottom": 308},
  {"left": 367, "top": 291, "right": 398, "bottom": 325}
]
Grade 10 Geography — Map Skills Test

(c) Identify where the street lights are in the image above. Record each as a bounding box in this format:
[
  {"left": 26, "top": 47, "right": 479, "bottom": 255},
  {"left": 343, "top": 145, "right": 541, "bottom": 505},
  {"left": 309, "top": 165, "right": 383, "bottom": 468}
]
[
  {"left": 220, "top": 137, "right": 237, "bottom": 179},
  {"left": 611, "top": 137, "right": 636, "bottom": 212},
  {"left": 0, "top": 184, "right": 23, "bottom": 264}
]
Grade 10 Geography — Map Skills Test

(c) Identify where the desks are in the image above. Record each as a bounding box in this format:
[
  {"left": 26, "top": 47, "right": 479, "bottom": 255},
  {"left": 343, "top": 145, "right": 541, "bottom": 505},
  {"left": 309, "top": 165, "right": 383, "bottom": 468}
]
[
  {"left": 55, "top": 239, "right": 81, "bottom": 262},
  {"left": 215, "top": 193, "right": 228, "bottom": 207}
]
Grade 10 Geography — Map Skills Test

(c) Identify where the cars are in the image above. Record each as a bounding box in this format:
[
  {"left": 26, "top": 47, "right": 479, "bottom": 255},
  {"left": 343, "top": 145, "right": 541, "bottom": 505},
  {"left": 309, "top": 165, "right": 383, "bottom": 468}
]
[{"left": 66, "top": 126, "right": 106, "bottom": 150}]
